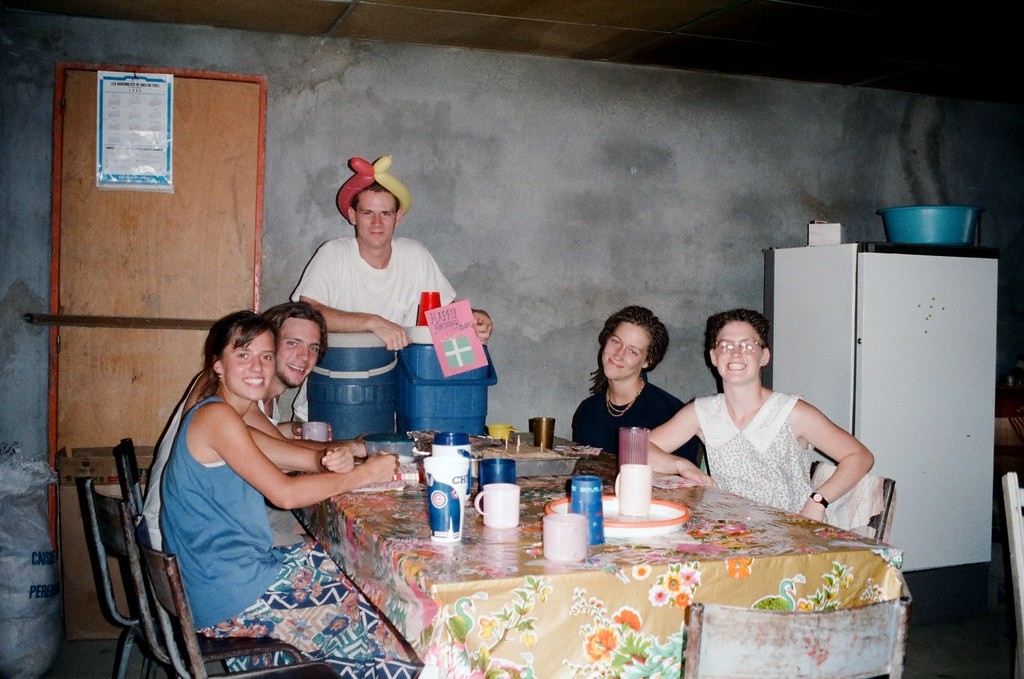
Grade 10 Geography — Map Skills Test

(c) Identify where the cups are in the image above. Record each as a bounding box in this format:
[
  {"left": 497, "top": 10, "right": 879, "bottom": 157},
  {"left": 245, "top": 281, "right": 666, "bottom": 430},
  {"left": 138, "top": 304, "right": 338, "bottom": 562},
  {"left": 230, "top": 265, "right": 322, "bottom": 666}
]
[
  {"left": 417, "top": 291, "right": 440, "bottom": 326},
  {"left": 479, "top": 458, "right": 516, "bottom": 490},
  {"left": 474, "top": 483, "right": 521, "bottom": 529},
  {"left": 570, "top": 475, "right": 604, "bottom": 545},
  {"left": 534, "top": 417, "right": 555, "bottom": 449},
  {"left": 543, "top": 514, "right": 588, "bottom": 564},
  {"left": 423, "top": 457, "right": 470, "bottom": 543},
  {"left": 486, "top": 423, "right": 512, "bottom": 440},
  {"left": 430, "top": 433, "right": 472, "bottom": 501},
  {"left": 301, "top": 422, "right": 328, "bottom": 442},
  {"left": 615, "top": 464, "right": 653, "bottom": 516},
  {"left": 618, "top": 427, "right": 649, "bottom": 468}
]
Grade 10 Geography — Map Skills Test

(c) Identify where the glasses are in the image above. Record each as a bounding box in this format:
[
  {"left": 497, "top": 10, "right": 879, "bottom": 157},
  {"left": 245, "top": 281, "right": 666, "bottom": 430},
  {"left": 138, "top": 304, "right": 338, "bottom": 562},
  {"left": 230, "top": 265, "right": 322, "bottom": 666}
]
[{"left": 714, "top": 337, "right": 764, "bottom": 355}]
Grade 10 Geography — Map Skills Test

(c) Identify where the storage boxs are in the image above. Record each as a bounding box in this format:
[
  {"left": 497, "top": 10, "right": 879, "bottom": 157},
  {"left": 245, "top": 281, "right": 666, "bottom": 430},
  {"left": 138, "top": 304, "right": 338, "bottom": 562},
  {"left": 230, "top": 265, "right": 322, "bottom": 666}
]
[
  {"left": 515, "top": 456, "right": 581, "bottom": 479},
  {"left": 54, "top": 442, "right": 154, "bottom": 640},
  {"left": 808, "top": 220, "right": 848, "bottom": 245}
]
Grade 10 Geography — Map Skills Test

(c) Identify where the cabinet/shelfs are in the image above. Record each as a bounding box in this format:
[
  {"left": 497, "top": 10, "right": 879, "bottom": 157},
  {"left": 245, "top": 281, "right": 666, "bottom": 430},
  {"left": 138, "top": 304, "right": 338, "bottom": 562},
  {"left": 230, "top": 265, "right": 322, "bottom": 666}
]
[{"left": 757, "top": 247, "right": 998, "bottom": 578}]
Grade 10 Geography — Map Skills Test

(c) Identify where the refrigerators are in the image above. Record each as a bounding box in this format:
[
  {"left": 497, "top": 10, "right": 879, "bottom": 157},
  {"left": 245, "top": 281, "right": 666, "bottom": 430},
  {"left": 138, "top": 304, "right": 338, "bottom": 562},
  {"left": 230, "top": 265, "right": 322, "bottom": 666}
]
[{"left": 761, "top": 243, "right": 999, "bottom": 571}]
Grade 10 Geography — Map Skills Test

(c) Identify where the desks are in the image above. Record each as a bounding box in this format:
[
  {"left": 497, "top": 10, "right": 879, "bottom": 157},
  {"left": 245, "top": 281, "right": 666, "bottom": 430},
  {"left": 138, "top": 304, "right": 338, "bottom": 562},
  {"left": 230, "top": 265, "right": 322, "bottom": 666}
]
[{"left": 289, "top": 431, "right": 911, "bottom": 679}]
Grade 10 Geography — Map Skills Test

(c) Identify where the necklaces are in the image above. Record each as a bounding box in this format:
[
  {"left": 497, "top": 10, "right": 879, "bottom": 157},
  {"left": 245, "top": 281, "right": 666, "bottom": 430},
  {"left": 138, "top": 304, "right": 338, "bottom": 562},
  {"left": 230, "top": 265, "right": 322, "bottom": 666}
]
[{"left": 606, "top": 381, "right": 644, "bottom": 416}]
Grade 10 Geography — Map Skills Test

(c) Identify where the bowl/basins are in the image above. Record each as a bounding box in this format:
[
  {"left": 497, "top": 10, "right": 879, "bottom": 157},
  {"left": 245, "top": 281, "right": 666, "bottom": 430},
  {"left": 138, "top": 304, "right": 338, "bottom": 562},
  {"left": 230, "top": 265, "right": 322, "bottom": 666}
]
[
  {"left": 876, "top": 205, "right": 988, "bottom": 244},
  {"left": 362, "top": 434, "right": 416, "bottom": 465}
]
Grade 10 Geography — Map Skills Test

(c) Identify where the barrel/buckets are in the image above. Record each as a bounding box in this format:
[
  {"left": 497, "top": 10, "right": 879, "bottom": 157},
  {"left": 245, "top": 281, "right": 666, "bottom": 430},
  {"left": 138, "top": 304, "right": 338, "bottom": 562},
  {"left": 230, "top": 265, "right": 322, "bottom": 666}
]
[
  {"left": 305, "top": 331, "right": 397, "bottom": 441},
  {"left": 398, "top": 326, "right": 498, "bottom": 440}
]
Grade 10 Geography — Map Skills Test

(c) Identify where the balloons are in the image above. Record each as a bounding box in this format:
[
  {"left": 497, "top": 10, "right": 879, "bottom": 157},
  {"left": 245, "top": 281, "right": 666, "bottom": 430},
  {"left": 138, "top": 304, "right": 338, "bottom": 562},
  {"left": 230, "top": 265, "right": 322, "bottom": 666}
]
[{"left": 337, "top": 156, "right": 412, "bottom": 218}]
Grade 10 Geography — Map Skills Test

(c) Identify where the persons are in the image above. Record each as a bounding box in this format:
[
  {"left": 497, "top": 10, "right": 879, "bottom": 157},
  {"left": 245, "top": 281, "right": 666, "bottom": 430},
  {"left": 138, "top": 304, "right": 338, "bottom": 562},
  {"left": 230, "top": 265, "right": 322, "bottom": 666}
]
[
  {"left": 572, "top": 305, "right": 699, "bottom": 465},
  {"left": 143, "top": 302, "right": 366, "bottom": 552},
  {"left": 648, "top": 309, "right": 875, "bottom": 523},
  {"left": 290, "top": 182, "right": 493, "bottom": 351},
  {"left": 160, "top": 310, "right": 424, "bottom": 679}
]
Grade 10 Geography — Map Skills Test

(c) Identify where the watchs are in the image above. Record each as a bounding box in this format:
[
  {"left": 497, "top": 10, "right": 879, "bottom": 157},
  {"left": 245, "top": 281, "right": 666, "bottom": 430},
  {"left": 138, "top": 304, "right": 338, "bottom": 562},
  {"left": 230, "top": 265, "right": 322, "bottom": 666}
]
[{"left": 810, "top": 493, "right": 829, "bottom": 508}]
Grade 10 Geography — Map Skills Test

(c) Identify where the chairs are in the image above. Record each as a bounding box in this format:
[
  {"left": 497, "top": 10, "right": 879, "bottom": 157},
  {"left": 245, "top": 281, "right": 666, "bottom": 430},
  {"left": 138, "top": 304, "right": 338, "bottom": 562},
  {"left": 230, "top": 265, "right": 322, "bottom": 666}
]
[
  {"left": 681, "top": 593, "right": 911, "bottom": 679},
  {"left": 1002, "top": 471, "right": 1024, "bottom": 677},
  {"left": 131, "top": 512, "right": 344, "bottom": 679},
  {"left": 111, "top": 437, "right": 145, "bottom": 517},
  {"left": 803, "top": 458, "right": 899, "bottom": 542},
  {"left": 80, "top": 474, "right": 303, "bottom": 679}
]
